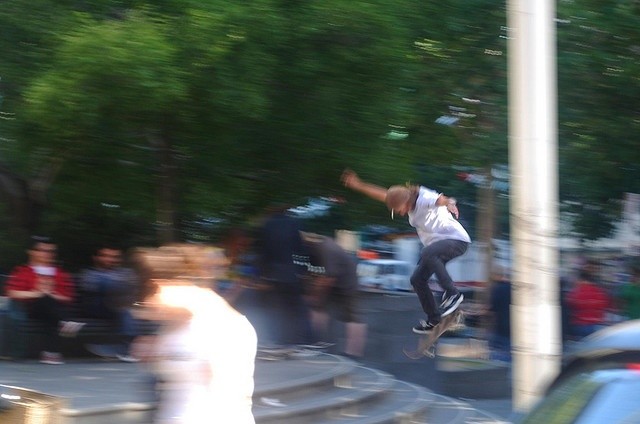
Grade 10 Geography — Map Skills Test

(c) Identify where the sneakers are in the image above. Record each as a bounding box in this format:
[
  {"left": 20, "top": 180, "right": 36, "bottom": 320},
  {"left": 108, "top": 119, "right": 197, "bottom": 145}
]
[
  {"left": 437, "top": 292, "right": 464, "bottom": 319},
  {"left": 412, "top": 320, "right": 441, "bottom": 335}
]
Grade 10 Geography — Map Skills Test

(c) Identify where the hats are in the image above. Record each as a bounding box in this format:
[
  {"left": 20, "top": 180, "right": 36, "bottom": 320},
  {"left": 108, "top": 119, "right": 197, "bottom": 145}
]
[{"left": 385, "top": 184, "right": 412, "bottom": 211}]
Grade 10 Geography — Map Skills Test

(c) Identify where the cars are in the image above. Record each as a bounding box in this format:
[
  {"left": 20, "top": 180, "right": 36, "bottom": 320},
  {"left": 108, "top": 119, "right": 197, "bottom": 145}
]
[{"left": 522, "top": 317, "right": 640, "bottom": 424}]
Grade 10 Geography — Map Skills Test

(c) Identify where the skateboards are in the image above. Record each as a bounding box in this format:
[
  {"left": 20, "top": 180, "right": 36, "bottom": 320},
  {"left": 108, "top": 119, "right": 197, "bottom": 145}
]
[{"left": 417, "top": 309, "right": 464, "bottom": 359}]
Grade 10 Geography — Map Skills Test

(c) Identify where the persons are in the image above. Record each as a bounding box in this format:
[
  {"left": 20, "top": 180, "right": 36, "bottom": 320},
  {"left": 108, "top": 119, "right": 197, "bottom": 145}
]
[
  {"left": 341, "top": 170, "right": 471, "bottom": 336},
  {"left": 133, "top": 244, "right": 258, "bottom": 424},
  {"left": 485, "top": 265, "right": 510, "bottom": 338},
  {"left": 564, "top": 269, "right": 607, "bottom": 337},
  {"left": 78, "top": 244, "right": 145, "bottom": 354},
  {"left": 298, "top": 217, "right": 366, "bottom": 360},
  {"left": 8, "top": 241, "right": 78, "bottom": 366}
]
